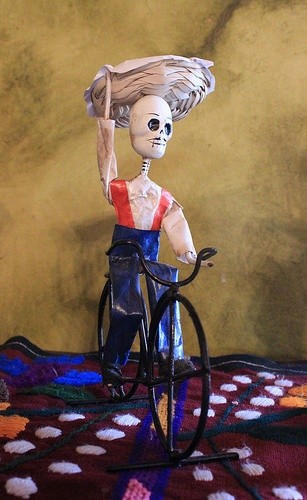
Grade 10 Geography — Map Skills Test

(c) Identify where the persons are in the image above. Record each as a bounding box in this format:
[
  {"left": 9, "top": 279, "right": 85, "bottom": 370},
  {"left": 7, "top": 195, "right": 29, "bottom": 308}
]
[{"left": 82, "top": 55, "right": 215, "bottom": 380}]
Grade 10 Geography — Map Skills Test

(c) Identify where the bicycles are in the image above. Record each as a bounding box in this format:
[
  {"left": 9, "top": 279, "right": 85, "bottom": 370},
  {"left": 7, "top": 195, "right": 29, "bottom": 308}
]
[{"left": 96, "top": 241, "right": 218, "bottom": 462}]
